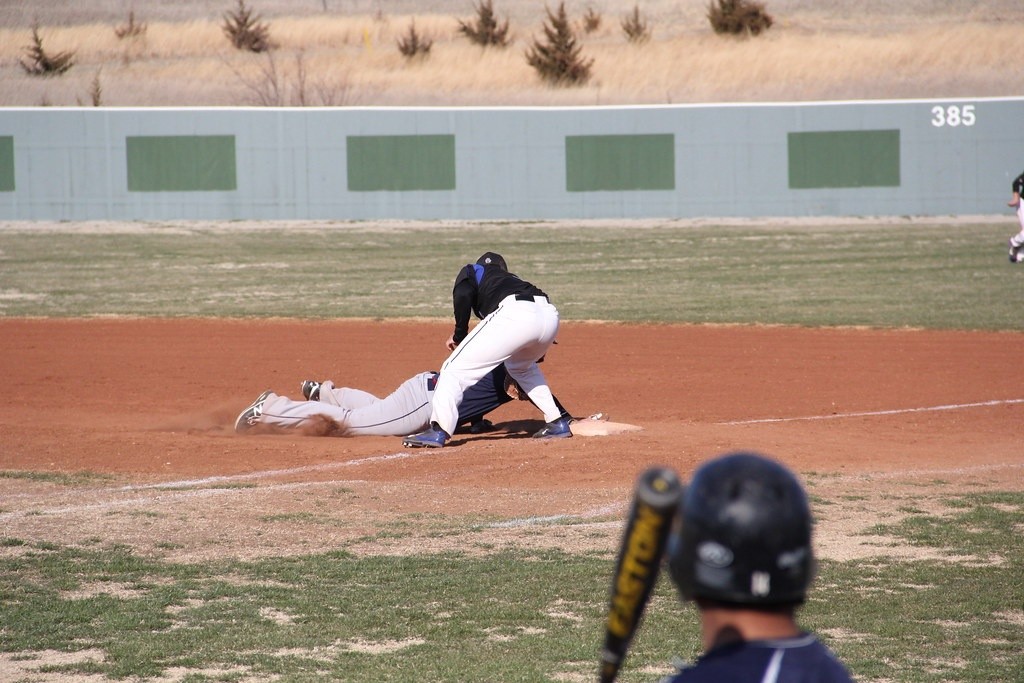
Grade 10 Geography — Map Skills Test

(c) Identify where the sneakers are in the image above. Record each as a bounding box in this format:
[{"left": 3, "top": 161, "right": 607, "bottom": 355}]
[
  {"left": 533, "top": 419, "right": 573, "bottom": 439},
  {"left": 401, "top": 430, "right": 446, "bottom": 448},
  {"left": 301, "top": 379, "right": 322, "bottom": 401},
  {"left": 234, "top": 390, "right": 272, "bottom": 432}
]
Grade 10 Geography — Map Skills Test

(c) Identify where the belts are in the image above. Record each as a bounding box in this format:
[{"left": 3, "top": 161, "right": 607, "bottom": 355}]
[
  {"left": 488, "top": 294, "right": 549, "bottom": 313},
  {"left": 428, "top": 371, "right": 437, "bottom": 390}
]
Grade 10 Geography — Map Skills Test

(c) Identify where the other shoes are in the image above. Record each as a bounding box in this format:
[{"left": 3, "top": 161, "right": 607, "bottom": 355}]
[{"left": 1009, "top": 237, "right": 1018, "bottom": 262}]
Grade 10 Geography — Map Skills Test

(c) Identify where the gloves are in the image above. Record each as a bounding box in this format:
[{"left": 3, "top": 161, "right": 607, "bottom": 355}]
[{"left": 588, "top": 413, "right": 609, "bottom": 422}]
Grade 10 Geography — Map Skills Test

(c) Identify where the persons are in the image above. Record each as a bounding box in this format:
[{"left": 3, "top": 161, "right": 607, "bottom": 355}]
[
  {"left": 234, "top": 341, "right": 609, "bottom": 436},
  {"left": 1008, "top": 173, "right": 1024, "bottom": 263},
  {"left": 659, "top": 455, "right": 857, "bottom": 683},
  {"left": 403, "top": 252, "right": 573, "bottom": 448}
]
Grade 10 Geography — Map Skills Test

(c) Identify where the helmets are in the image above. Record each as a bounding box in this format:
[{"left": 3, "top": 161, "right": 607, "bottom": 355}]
[
  {"left": 476, "top": 252, "right": 507, "bottom": 271},
  {"left": 668, "top": 454, "right": 811, "bottom": 604}
]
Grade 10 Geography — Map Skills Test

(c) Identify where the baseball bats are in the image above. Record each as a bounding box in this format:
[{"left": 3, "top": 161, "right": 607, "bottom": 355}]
[{"left": 598, "top": 467, "right": 684, "bottom": 683}]
